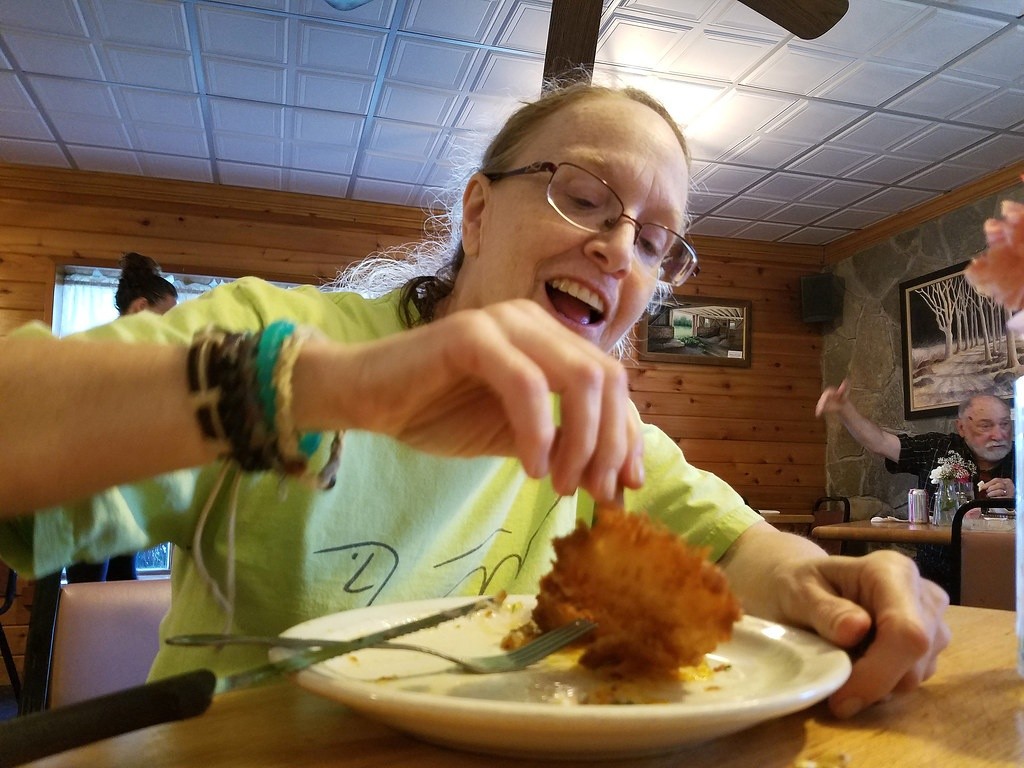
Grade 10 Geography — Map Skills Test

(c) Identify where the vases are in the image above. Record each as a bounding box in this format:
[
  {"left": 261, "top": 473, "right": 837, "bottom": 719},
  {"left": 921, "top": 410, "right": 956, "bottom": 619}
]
[{"left": 934, "top": 482, "right": 974, "bottom": 525}]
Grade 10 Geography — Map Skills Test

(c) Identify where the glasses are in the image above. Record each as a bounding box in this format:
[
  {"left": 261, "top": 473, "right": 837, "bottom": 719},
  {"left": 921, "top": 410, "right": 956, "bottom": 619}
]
[
  {"left": 486, "top": 160, "right": 701, "bottom": 287},
  {"left": 961, "top": 420, "right": 1014, "bottom": 431}
]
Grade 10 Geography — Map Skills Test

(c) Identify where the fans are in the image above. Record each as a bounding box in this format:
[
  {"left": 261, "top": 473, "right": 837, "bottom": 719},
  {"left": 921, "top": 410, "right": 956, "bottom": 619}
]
[{"left": 323, "top": 0, "right": 854, "bottom": 98}]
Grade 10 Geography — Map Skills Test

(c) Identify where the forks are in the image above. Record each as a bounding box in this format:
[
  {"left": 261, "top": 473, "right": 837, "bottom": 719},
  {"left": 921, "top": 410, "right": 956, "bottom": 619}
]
[{"left": 162, "top": 614, "right": 598, "bottom": 673}]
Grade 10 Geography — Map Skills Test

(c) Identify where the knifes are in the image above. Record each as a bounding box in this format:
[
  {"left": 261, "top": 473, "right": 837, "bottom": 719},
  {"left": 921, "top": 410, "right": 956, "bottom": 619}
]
[{"left": 0, "top": 592, "right": 497, "bottom": 767}]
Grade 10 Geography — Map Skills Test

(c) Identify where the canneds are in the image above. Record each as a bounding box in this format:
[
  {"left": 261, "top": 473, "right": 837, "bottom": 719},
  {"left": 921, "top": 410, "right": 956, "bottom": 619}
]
[{"left": 908, "top": 488, "right": 929, "bottom": 524}]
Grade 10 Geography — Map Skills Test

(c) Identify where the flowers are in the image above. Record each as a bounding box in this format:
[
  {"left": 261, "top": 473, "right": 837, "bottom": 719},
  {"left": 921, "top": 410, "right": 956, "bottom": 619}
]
[{"left": 930, "top": 449, "right": 977, "bottom": 515}]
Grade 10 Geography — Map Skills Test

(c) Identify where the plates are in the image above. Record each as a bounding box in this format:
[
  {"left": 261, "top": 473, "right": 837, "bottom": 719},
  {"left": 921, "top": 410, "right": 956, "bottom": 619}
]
[{"left": 267, "top": 594, "right": 851, "bottom": 762}]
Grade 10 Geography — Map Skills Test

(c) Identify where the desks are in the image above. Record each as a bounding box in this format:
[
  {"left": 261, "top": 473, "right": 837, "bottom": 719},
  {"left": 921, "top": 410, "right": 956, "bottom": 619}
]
[
  {"left": 757, "top": 514, "right": 814, "bottom": 527},
  {"left": 809, "top": 517, "right": 960, "bottom": 558},
  {"left": 13, "top": 606, "right": 1023, "bottom": 768}
]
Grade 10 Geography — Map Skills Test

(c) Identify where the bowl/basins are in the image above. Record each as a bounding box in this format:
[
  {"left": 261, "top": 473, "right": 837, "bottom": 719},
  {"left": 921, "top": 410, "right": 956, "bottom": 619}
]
[{"left": 950, "top": 514, "right": 1016, "bottom": 531}]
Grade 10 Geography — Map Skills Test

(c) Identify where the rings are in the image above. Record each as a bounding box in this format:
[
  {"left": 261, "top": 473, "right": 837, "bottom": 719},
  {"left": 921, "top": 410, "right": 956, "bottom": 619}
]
[{"left": 1002, "top": 489, "right": 1007, "bottom": 496}]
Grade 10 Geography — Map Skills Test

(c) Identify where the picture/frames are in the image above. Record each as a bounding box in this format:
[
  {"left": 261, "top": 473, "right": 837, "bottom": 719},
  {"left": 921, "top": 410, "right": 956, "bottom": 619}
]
[
  {"left": 638, "top": 291, "right": 751, "bottom": 367},
  {"left": 899, "top": 259, "right": 1024, "bottom": 421}
]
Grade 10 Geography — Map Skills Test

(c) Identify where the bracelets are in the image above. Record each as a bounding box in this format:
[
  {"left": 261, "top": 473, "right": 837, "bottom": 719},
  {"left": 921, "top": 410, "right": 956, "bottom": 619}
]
[{"left": 186, "top": 320, "right": 344, "bottom": 488}]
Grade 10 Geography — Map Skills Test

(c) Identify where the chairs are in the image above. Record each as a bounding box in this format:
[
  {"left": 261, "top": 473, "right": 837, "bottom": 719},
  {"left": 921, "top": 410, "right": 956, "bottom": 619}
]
[
  {"left": 807, "top": 498, "right": 850, "bottom": 555},
  {"left": 949, "top": 498, "right": 1016, "bottom": 608},
  {"left": 21, "top": 558, "right": 175, "bottom": 710}
]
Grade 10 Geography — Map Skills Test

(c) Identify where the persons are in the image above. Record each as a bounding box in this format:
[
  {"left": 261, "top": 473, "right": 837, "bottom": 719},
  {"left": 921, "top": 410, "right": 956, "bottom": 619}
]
[
  {"left": 0, "top": 85, "right": 950, "bottom": 717},
  {"left": 816, "top": 378, "right": 1018, "bottom": 595},
  {"left": 114, "top": 252, "right": 175, "bottom": 319}
]
